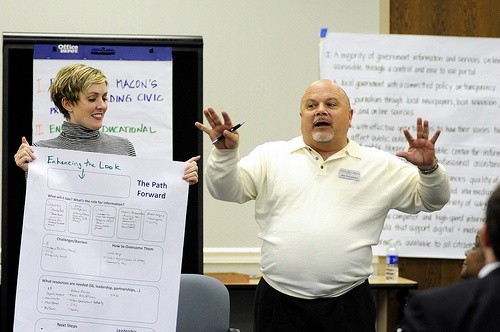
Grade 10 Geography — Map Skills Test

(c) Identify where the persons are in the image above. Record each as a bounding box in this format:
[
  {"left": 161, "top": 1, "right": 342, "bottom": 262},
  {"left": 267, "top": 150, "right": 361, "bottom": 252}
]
[
  {"left": 194, "top": 80, "right": 451, "bottom": 331},
  {"left": 14, "top": 64, "right": 201, "bottom": 185},
  {"left": 402, "top": 183, "right": 500, "bottom": 332}
]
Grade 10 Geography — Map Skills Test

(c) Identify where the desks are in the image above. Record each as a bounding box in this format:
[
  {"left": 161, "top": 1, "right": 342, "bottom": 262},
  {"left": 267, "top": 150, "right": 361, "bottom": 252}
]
[{"left": 203, "top": 274, "right": 419, "bottom": 332}]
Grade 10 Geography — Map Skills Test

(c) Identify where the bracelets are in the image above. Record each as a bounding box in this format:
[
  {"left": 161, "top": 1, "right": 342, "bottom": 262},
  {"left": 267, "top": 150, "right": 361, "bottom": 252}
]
[{"left": 417, "top": 156, "right": 438, "bottom": 173}]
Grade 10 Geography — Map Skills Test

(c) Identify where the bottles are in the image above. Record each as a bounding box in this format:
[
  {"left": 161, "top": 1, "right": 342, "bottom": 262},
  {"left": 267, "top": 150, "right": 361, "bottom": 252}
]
[{"left": 386, "top": 240, "right": 398, "bottom": 283}]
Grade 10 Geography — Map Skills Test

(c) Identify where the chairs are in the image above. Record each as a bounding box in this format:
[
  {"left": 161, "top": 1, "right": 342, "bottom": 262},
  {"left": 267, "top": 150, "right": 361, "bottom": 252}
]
[{"left": 176, "top": 275, "right": 230, "bottom": 332}]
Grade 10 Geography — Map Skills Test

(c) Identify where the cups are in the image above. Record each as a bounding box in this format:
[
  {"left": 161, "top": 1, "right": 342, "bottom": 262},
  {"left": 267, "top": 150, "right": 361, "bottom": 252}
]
[{"left": 371, "top": 256, "right": 379, "bottom": 279}]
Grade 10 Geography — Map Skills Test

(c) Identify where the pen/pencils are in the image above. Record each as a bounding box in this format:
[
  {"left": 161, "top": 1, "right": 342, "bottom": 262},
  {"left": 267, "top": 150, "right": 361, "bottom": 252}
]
[{"left": 209, "top": 121, "right": 244, "bottom": 147}]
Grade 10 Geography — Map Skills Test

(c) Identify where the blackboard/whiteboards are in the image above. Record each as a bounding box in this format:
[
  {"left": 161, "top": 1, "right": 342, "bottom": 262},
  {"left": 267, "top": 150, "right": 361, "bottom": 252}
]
[{"left": 0, "top": 32, "right": 206, "bottom": 332}]
